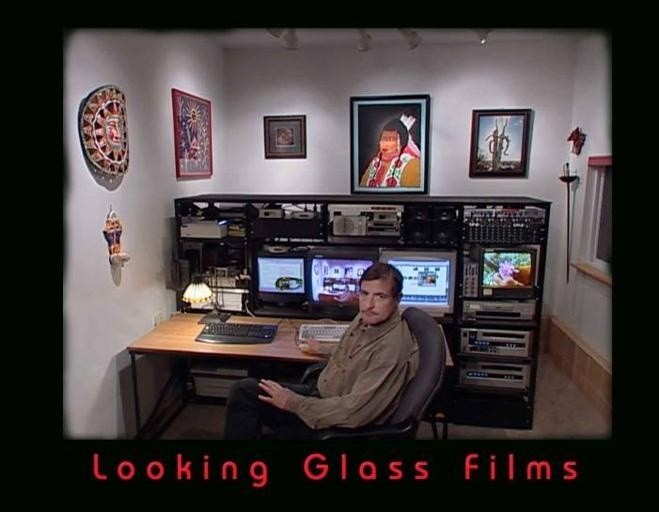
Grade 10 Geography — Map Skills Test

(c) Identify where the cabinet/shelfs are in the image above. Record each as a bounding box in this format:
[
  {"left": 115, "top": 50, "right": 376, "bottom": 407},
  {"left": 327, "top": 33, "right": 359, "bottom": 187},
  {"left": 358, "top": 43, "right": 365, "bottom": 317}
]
[{"left": 173, "top": 194, "right": 554, "bottom": 431}]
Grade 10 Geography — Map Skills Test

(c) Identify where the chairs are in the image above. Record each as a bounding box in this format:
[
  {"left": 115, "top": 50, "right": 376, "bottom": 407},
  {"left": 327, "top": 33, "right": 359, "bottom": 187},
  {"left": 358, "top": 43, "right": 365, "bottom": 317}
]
[{"left": 294, "top": 307, "right": 447, "bottom": 440}]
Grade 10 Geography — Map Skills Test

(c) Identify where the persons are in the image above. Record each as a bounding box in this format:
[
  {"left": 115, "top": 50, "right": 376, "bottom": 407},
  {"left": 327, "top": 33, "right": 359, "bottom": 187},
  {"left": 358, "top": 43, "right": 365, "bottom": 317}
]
[
  {"left": 358, "top": 118, "right": 423, "bottom": 190},
  {"left": 220, "top": 262, "right": 422, "bottom": 438}
]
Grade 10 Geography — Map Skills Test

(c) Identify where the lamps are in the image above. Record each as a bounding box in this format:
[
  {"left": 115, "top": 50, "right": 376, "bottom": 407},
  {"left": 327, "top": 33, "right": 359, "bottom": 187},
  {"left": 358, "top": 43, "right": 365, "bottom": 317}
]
[
  {"left": 264, "top": 25, "right": 488, "bottom": 52},
  {"left": 180, "top": 273, "right": 232, "bottom": 325}
]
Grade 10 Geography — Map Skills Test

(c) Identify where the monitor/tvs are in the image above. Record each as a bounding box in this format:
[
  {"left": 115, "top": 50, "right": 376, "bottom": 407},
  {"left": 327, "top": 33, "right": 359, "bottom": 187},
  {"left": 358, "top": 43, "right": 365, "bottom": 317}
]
[
  {"left": 481, "top": 247, "right": 537, "bottom": 290},
  {"left": 251, "top": 255, "right": 308, "bottom": 320},
  {"left": 308, "top": 248, "right": 380, "bottom": 321},
  {"left": 378, "top": 247, "right": 457, "bottom": 319}
]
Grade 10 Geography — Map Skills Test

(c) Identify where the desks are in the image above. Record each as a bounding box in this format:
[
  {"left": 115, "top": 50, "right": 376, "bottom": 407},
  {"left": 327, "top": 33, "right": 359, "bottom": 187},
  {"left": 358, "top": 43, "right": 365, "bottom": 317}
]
[{"left": 126, "top": 310, "right": 456, "bottom": 440}]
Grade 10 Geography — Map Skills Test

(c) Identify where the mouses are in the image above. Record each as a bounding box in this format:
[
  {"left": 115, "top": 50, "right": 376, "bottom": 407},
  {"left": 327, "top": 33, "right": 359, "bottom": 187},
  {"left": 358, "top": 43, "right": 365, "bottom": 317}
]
[{"left": 299, "top": 341, "right": 307, "bottom": 350}]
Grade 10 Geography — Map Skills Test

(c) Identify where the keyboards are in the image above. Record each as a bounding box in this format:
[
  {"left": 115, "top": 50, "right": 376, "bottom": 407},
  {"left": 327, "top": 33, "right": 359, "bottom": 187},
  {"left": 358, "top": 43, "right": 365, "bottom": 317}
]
[
  {"left": 195, "top": 322, "right": 277, "bottom": 345},
  {"left": 299, "top": 324, "right": 351, "bottom": 343}
]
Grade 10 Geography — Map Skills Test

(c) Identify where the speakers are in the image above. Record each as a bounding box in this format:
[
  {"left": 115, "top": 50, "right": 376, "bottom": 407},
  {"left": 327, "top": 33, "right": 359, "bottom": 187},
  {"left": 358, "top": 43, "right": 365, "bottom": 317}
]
[
  {"left": 406, "top": 204, "right": 431, "bottom": 246},
  {"left": 432, "top": 204, "right": 458, "bottom": 246}
]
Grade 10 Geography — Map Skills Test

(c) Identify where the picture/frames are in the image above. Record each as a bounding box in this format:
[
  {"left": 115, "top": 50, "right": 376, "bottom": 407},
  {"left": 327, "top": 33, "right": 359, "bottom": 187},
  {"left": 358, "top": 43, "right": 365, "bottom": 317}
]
[
  {"left": 349, "top": 94, "right": 432, "bottom": 194},
  {"left": 171, "top": 88, "right": 213, "bottom": 177},
  {"left": 263, "top": 114, "right": 306, "bottom": 159},
  {"left": 469, "top": 108, "right": 532, "bottom": 178}
]
